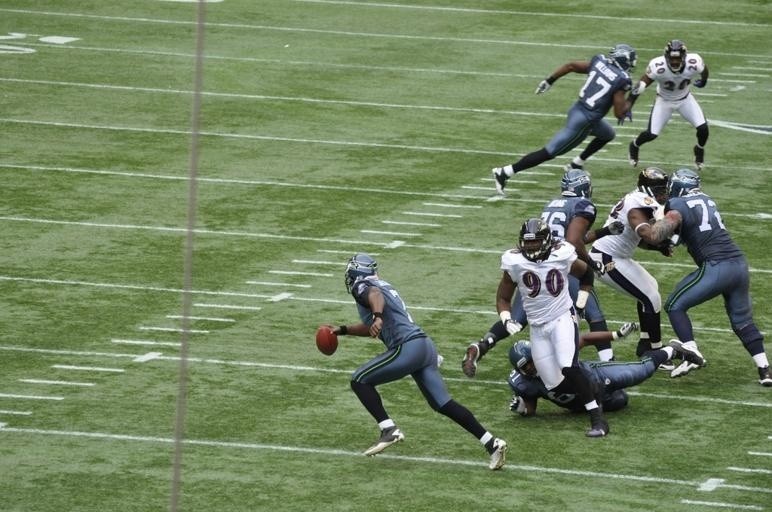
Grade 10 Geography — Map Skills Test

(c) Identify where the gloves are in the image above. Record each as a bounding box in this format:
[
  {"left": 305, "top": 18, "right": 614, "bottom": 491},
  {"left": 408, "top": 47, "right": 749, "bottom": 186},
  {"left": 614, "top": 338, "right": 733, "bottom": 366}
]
[
  {"left": 611, "top": 322, "right": 639, "bottom": 341},
  {"left": 535, "top": 77, "right": 554, "bottom": 95},
  {"left": 510, "top": 394, "right": 527, "bottom": 417},
  {"left": 694, "top": 78, "right": 708, "bottom": 88},
  {"left": 660, "top": 238, "right": 675, "bottom": 258},
  {"left": 595, "top": 221, "right": 625, "bottom": 241},
  {"left": 500, "top": 310, "right": 523, "bottom": 336},
  {"left": 628, "top": 81, "right": 646, "bottom": 105},
  {"left": 574, "top": 305, "right": 585, "bottom": 322},
  {"left": 617, "top": 108, "right": 635, "bottom": 126},
  {"left": 588, "top": 258, "right": 606, "bottom": 276}
]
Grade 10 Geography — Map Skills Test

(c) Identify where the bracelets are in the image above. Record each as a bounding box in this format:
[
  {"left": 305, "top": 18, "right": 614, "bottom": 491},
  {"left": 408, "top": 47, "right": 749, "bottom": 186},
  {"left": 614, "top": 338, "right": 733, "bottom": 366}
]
[
  {"left": 372, "top": 312, "right": 384, "bottom": 321},
  {"left": 340, "top": 325, "right": 346, "bottom": 334}
]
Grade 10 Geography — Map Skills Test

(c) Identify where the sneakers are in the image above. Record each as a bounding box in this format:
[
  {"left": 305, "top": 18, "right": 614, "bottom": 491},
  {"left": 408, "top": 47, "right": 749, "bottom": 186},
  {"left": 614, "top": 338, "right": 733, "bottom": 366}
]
[
  {"left": 636, "top": 337, "right": 707, "bottom": 379},
  {"left": 489, "top": 437, "right": 507, "bottom": 470},
  {"left": 492, "top": 167, "right": 511, "bottom": 196},
  {"left": 629, "top": 138, "right": 640, "bottom": 166},
  {"left": 757, "top": 365, "right": 772, "bottom": 386},
  {"left": 584, "top": 400, "right": 609, "bottom": 438},
  {"left": 363, "top": 425, "right": 407, "bottom": 456},
  {"left": 693, "top": 144, "right": 705, "bottom": 172},
  {"left": 461, "top": 343, "right": 480, "bottom": 378},
  {"left": 564, "top": 163, "right": 591, "bottom": 178}
]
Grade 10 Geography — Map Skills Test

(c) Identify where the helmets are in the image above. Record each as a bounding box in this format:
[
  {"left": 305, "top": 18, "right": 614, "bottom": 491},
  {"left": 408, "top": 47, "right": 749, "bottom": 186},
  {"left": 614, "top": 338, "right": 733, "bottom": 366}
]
[
  {"left": 562, "top": 169, "right": 592, "bottom": 198},
  {"left": 664, "top": 40, "right": 687, "bottom": 74},
  {"left": 610, "top": 44, "right": 637, "bottom": 73},
  {"left": 344, "top": 253, "right": 377, "bottom": 294},
  {"left": 519, "top": 218, "right": 552, "bottom": 262},
  {"left": 509, "top": 340, "right": 537, "bottom": 378},
  {"left": 637, "top": 167, "right": 670, "bottom": 197},
  {"left": 666, "top": 168, "right": 700, "bottom": 197}
]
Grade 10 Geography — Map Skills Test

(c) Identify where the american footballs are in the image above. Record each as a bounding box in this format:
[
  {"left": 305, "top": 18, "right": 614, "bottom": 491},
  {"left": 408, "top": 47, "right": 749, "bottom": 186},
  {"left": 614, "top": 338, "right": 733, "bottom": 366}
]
[{"left": 317, "top": 327, "right": 338, "bottom": 355}]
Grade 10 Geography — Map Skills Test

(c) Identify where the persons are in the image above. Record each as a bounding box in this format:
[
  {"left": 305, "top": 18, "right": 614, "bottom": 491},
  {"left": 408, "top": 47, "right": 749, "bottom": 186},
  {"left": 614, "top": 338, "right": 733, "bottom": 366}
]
[
  {"left": 492, "top": 41, "right": 708, "bottom": 194},
  {"left": 322, "top": 254, "right": 507, "bottom": 469},
  {"left": 462, "top": 166, "right": 772, "bottom": 437}
]
